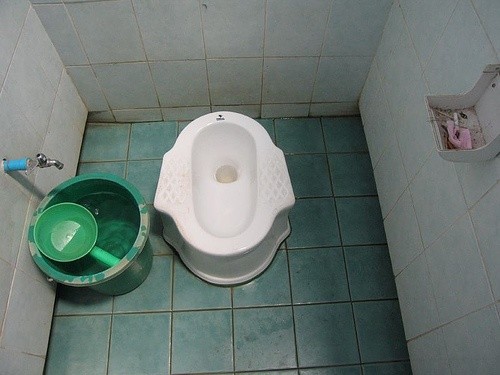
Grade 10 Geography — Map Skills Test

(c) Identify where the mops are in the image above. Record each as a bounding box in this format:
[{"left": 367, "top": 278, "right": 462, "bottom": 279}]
[{"left": 153, "top": 110, "right": 297, "bottom": 285}]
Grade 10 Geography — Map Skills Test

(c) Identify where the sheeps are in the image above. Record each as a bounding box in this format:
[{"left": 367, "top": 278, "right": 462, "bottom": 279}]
[{"left": 35, "top": 152, "right": 64, "bottom": 170}]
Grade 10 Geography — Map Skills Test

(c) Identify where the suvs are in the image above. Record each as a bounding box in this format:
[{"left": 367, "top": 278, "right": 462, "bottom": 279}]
[{"left": 27, "top": 172, "right": 154, "bottom": 297}]
